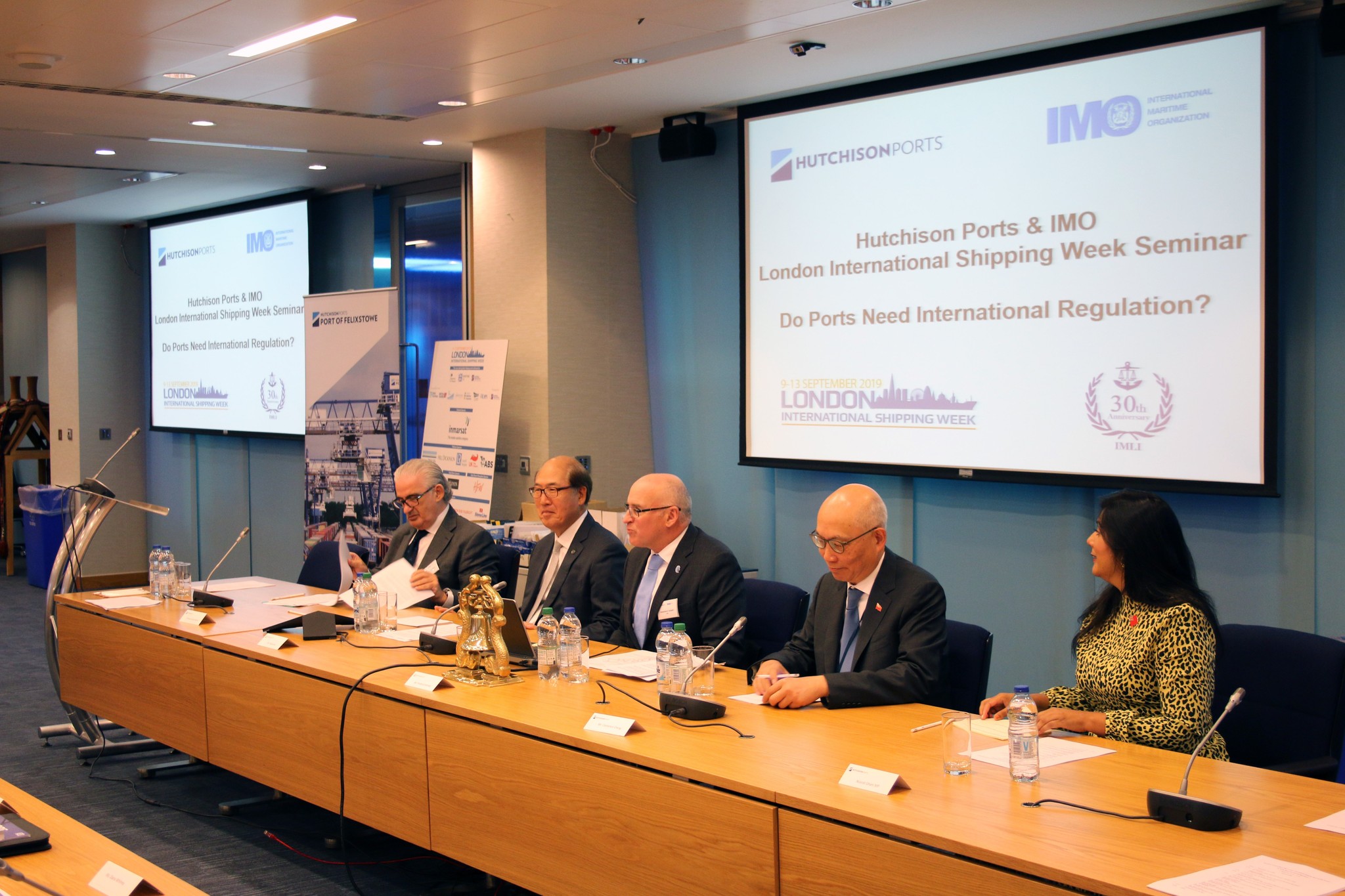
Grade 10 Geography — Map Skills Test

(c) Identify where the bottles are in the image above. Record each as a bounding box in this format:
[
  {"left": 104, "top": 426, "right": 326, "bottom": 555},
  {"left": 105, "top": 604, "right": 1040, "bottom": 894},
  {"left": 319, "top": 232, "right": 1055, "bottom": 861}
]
[
  {"left": 1007, "top": 685, "right": 1039, "bottom": 782},
  {"left": 655, "top": 621, "right": 675, "bottom": 696},
  {"left": 560, "top": 607, "right": 582, "bottom": 677},
  {"left": 358, "top": 573, "right": 379, "bottom": 634},
  {"left": 353, "top": 572, "right": 364, "bottom": 632},
  {"left": 667, "top": 623, "right": 692, "bottom": 697},
  {"left": 536, "top": 607, "right": 560, "bottom": 680},
  {"left": 148, "top": 544, "right": 161, "bottom": 596},
  {"left": 157, "top": 546, "right": 176, "bottom": 598}
]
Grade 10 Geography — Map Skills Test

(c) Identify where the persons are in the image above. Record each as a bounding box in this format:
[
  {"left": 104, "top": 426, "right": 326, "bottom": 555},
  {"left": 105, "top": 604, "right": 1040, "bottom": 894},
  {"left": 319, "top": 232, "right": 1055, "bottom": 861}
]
[
  {"left": 605, "top": 473, "right": 750, "bottom": 670},
  {"left": 751, "top": 483, "right": 947, "bottom": 710},
  {"left": 979, "top": 489, "right": 1230, "bottom": 762},
  {"left": 347, "top": 458, "right": 502, "bottom": 613},
  {"left": 520, "top": 455, "right": 629, "bottom": 643}
]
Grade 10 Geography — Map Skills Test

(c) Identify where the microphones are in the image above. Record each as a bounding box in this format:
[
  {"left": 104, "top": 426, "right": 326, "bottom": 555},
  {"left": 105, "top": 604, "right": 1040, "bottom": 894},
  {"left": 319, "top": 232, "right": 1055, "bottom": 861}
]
[
  {"left": 659, "top": 616, "right": 748, "bottom": 721},
  {"left": 193, "top": 527, "right": 250, "bottom": 607},
  {"left": 419, "top": 581, "right": 508, "bottom": 655},
  {"left": 82, "top": 427, "right": 141, "bottom": 499},
  {"left": 1146, "top": 687, "right": 1246, "bottom": 832}
]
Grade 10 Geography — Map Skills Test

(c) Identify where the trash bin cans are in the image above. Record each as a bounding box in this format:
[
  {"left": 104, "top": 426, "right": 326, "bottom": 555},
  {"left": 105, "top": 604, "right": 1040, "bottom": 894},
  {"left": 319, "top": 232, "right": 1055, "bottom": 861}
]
[{"left": 17, "top": 483, "right": 76, "bottom": 591}]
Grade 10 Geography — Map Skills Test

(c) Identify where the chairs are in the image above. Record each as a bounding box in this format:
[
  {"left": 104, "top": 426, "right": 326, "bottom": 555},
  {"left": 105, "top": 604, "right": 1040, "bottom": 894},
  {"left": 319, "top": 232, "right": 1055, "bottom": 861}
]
[
  {"left": 738, "top": 577, "right": 811, "bottom": 668},
  {"left": 501, "top": 545, "right": 522, "bottom": 600},
  {"left": 1210, "top": 621, "right": 1344, "bottom": 786},
  {"left": 937, "top": 619, "right": 995, "bottom": 715},
  {"left": 296, "top": 542, "right": 370, "bottom": 591}
]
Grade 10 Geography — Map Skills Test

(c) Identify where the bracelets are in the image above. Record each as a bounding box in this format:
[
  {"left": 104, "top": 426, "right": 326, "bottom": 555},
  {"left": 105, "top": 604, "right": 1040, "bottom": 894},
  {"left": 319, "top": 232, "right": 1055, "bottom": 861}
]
[{"left": 436, "top": 589, "right": 448, "bottom": 606}]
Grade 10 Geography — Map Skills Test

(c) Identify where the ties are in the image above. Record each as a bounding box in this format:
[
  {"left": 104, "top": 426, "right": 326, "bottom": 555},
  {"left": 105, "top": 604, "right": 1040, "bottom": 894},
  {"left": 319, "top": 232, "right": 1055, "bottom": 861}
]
[
  {"left": 526, "top": 540, "right": 563, "bottom": 623},
  {"left": 839, "top": 587, "right": 864, "bottom": 672},
  {"left": 633, "top": 555, "right": 664, "bottom": 650},
  {"left": 402, "top": 529, "right": 430, "bottom": 567}
]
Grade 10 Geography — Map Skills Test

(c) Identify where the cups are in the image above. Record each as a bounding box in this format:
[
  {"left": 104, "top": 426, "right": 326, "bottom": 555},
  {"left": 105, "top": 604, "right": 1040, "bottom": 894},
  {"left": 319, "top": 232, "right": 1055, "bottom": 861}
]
[
  {"left": 377, "top": 591, "right": 388, "bottom": 630},
  {"left": 174, "top": 561, "right": 191, "bottom": 596},
  {"left": 378, "top": 592, "right": 398, "bottom": 632},
  {"left": 691, "top": 646, "right": 715, "bottom": 696},
  {"left": 941, "top": 711, "right": 972, "bottom": 776},
  {"left": 566, "top": 636, "right": 591, "bottom": 684}
]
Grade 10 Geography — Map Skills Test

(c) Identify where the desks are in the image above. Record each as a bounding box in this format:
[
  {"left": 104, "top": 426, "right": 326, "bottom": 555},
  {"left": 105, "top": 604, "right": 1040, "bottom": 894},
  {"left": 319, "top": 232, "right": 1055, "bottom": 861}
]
[
  {"left": 52, "top": 577, "right": 1345, "bottom": 896},
  {"left": 0, "top": 776, "right": 212, "bottom": 896}
]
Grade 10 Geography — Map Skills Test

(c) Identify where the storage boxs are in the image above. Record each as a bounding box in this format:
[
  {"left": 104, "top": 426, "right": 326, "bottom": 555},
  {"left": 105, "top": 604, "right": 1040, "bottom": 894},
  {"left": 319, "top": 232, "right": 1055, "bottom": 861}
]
[{"left": 478, "top": 497, "right": 636, "bottom": 577}]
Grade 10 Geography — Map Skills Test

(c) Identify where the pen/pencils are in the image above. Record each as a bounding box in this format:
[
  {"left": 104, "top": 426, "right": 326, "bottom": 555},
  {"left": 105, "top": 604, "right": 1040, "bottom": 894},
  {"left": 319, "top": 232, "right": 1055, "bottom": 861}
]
[
  {"left": 271, "top": 593, "right": 305, "bottom": 601},
  {"left": 288, "top": 610, "right": 305, "bottom": 615},
  {"left": 911, "top": 721, "right": 943, "bottom": 732},
  {"left": 756, "top": 673, "right": 800, "bottom": 678}
]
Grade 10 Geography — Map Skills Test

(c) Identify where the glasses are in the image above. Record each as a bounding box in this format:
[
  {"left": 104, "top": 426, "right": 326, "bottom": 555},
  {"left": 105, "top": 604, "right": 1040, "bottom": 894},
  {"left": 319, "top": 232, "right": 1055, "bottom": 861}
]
[
  {"left": 623, "top": 504, "right": 681, "bottom": 519},
  {"left": 392, "top": 486, "right": 435, "bottom": 510},
  {"left": 529, "top": 485, "right": 572, "bottom": 499},
  {"left": 809, "top": 527, "right": 878, "bottom": 553}
]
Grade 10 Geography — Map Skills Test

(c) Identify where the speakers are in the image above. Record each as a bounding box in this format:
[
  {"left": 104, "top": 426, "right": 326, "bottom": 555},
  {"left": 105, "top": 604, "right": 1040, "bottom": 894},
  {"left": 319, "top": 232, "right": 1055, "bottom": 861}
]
[{"left": 656, "top": 124, "right": 716, "bottom": 161}]
